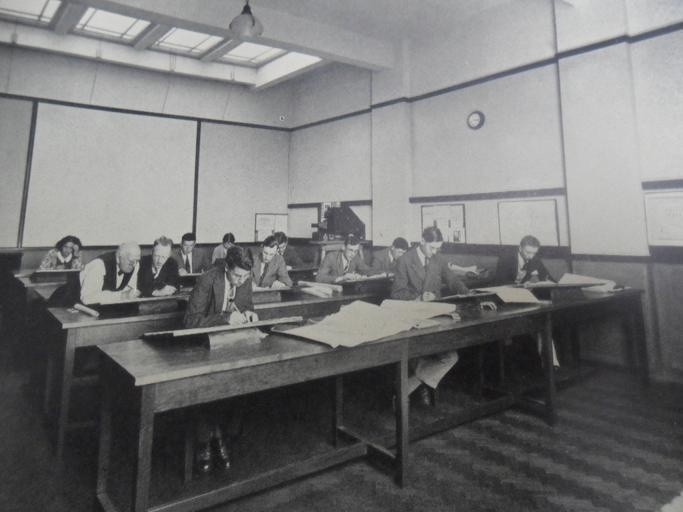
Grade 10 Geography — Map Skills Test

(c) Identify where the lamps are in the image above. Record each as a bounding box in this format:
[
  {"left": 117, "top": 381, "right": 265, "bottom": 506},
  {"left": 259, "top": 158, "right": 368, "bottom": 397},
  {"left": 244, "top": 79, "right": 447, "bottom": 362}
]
[{"left": 229, "top": 2, "right": 263, "bottom": 42}]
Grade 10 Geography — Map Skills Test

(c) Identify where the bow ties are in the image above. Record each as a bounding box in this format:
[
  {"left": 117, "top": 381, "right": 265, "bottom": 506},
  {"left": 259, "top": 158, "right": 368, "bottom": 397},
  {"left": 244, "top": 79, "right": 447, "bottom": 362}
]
[{"left": 118, "top": 270, "right": 123, "bottom": 275}]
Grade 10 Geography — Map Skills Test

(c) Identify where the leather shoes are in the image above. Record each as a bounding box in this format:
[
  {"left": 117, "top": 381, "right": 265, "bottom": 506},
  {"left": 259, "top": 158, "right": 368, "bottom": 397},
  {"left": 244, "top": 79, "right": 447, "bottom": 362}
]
[
  {"left": 196, "top": 440, "right": 214, "bottom": 474},
  {"left": 213, "top": 436, "right": 232, "bottom": 471},
  {"left": 390, "top": 395, "right": 396, "bottom": 414},
  {"left": 543, "top": 366, "right": 569, "bottom": 382},
  {"left": 415, "top": 387, "right": 430, "bottom": 408}
]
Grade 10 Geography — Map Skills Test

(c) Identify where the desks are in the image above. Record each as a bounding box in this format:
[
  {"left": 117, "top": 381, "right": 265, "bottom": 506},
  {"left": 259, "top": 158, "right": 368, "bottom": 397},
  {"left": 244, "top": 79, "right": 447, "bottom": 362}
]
[
  {"left": 11, "top": 266, "right": 317, "bottom": 300},
  {"left": 43, "top": 277, "right": 498, "bottom": 461},
  {"left": 308, "top": 240, "right": 372, "bottom": 269},
  {"left": 95, "top": 283, "right": 645, "bottom": 511}
]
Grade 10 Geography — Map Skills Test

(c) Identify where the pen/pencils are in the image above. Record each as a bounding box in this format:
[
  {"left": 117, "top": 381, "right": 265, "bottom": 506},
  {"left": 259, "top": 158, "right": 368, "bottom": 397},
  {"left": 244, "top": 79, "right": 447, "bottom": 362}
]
[
  {"left": 126, "top": 284, "right": 144, "bottom": 297},
  {"left": 232, "top": 303, "right": 240, "bottom": 313}
]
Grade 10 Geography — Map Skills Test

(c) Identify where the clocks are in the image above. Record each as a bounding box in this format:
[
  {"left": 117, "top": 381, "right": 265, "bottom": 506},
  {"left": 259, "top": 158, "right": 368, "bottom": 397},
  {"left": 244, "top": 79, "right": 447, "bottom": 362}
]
[{"left": 467, "top": 112, "right": 485, "bottom": 129}]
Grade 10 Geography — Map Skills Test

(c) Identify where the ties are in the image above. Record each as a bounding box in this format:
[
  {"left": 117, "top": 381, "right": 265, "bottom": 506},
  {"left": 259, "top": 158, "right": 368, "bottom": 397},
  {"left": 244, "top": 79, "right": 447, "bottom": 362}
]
[
  {"left": 186, "top": 255, "right": 190, "bottom": 273},
  {"left": 425, "top": 258, "right": 428, "bottom": 271},
  {"left": 259, "top": 263, "right": 268, "bottom": 286},
  {"left": 344, "top": 260, "right": 349, "bottom": 273},
  {"left": 154, "top": 268, "right": 159, "bottom": 279},
  {"left": 392, "top": 260, "right": 396, "bottom": 265},
  {"left": 522, "top": 261, "right": 529, "bottom": 271},
  {"left": 225, "top": 283, "right": 234, "bottom": 312}
]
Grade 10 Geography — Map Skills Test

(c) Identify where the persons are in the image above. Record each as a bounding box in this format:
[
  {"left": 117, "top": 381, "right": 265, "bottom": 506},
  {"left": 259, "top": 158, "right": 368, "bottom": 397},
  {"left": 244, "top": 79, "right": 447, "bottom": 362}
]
[
  {"left": 207, "top": 233, "right": 235, "bottom": 263},
  {"left": 183, "top": 246, "right": 260, "bottom": 475},
  {"left": 36, "top": 235, "right": 82, "bottom": 271},
  {"left": 46, "top": 241, "right": 142, "bottom": 306},
  {"left": 252, "top": 236, "right": 293, "bottom": 291},
  {"left": 314, "top": 237, "right": 372, "bottom": 284},
  {"left": 389, "top": 226, "right": 497, "bottom": 413},
  {"left": 372, "top": 237, "right": 409, "bottom": 275},
  {"left": 275, "top": 231, "right": 305, "bottom": 271},
  {"left": 174, "top": 232, "right": 213, "bottom": 276},
  {"left": 138, "top": 236, "right": 181, "bottom": 297},
  {"left": 493, "top": 235, "right": 560, "bottom": 372}
]
